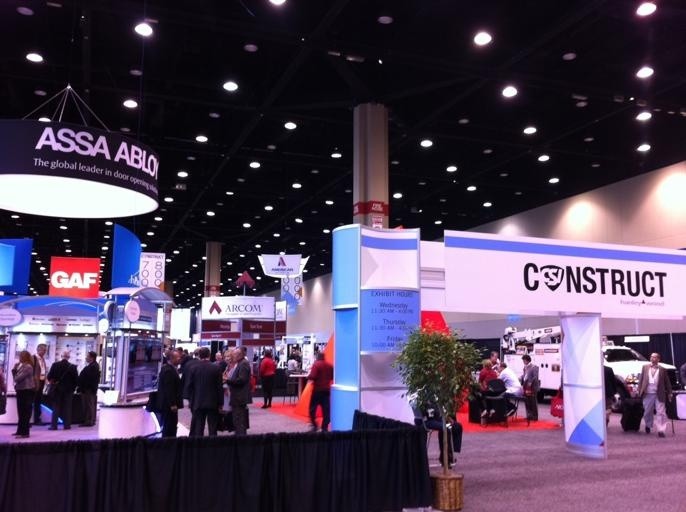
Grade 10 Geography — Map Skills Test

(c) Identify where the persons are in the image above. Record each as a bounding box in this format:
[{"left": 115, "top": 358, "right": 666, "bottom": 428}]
[
  {"left": 470, "top": 350, "right": 524, "bottom": 422},
  {"left": 307, "top": 352, "right": 333, "bottom": 432},
  {"left": 411, "top": 389, "right": 462, "bottom": 469},
  {"left": 11, "top": 351, "right": 36, "bottom": 439},
  {"left": 521, "top": 355, "right": 538, "bottom": 421},
  {"left": 637, "top": 352, "right": 673, "bottom": 437},
  {"left": 47, "top": 351, "right": 79, "bottom": 431},
  {"left": 76, "top": 351, "right": 100, "bottom": 427},
  {"left": 146, "top": 343, "right": 302, "bottom": 437},
  {"left": 31, "top": 344, "right": 48, "bottom": 425}
]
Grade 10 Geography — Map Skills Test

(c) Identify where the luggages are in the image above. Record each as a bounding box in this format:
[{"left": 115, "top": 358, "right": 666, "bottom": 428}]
[{"left": 619, "top": 395, "right": 645, "bottom": 434}]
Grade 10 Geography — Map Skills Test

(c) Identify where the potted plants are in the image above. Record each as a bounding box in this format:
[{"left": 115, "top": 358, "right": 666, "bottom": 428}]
[{"left": 390, "top": 315, "right": 489, "bottom": 512}]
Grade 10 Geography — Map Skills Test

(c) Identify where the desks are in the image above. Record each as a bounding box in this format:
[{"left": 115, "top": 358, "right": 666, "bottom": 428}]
[{"left": 289, "top": 372, "right": 308, "bottom": 401}]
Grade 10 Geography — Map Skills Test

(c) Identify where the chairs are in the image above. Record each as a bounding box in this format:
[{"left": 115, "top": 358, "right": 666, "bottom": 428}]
[{"left": 466, "top": 378, "right": 529, "bottom": 424}]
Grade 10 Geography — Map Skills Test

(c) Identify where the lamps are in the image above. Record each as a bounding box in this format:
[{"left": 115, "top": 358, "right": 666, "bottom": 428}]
[{"left": 0, "top": 82, "right": 160, "bottom": 219}]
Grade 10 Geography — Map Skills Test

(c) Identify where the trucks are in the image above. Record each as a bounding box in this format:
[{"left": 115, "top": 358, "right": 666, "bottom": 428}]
[{"left": 532, "top": 339, "right": 678, "bottom": 413}]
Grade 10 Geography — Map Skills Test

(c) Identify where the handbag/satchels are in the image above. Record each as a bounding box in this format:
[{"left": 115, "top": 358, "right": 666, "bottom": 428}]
[
  {"left": 144, "top": 389, "right": 158, "bottom": 414},
  {"left": 550, "top": 387, "right": 564, "bottom": 418}
]
